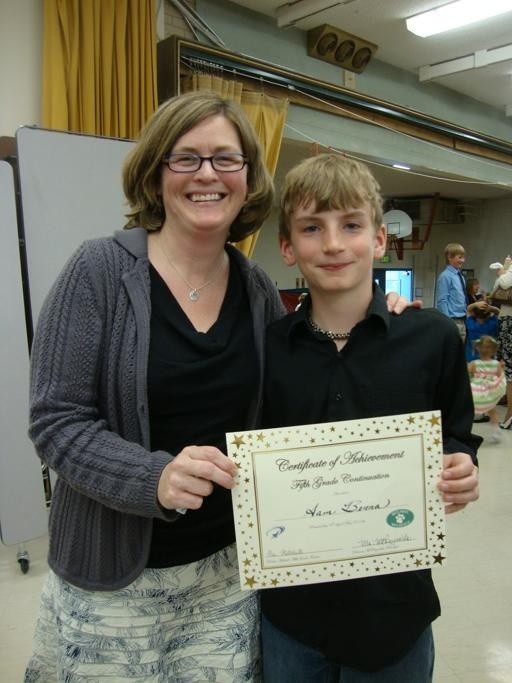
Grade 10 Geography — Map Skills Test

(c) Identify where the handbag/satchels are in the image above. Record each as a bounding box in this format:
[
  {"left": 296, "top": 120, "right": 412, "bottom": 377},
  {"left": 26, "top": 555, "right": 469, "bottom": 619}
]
[{"left": 487, "top": 285, "right": 512, "bottom": 306}]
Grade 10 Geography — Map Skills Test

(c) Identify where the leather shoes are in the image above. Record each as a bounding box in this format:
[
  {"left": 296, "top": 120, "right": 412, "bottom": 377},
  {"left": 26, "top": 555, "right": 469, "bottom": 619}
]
[
  {"left": 474, "top": 416, "right": 490, "bottom": 423},
  {"left": 498, "top": 416, "right": 511, "bottom": 429}
]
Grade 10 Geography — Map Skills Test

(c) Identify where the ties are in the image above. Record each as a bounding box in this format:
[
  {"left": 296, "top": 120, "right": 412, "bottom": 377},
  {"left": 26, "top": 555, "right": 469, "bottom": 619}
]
[{"left": 457, "top": 272, "right": 469, "bottom": 306}]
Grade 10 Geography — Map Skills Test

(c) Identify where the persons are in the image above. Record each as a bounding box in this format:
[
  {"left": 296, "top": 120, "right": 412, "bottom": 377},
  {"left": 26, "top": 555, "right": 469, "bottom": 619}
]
[
  {"left": 230, "top": 154, "right": 484, "bottom": 683},
  {"left": 435, "top": 243, "right": 512, "bottom": 429},
  {"left": 23, "top": 92, "right": 423, "bottom": 683}
]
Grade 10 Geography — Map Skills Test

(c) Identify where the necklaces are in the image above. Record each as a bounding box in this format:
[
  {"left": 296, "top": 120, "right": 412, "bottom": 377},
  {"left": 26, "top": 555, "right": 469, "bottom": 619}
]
[
  {"left": 159, "top": 241, "right": 222, "bottom": 301},
  {"left": 307, "top": 315, "right": 351, "bottom": 339}
]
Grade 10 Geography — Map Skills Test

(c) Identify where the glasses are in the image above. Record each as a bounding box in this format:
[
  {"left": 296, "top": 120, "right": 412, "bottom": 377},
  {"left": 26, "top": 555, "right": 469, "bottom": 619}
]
[{"left": 161, "top": 151, "right": 249, "bottom": 174}]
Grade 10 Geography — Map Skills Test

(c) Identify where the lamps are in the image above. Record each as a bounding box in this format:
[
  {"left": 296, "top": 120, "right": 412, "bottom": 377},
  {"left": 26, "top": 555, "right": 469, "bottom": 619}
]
[{"left": 305, "top": 19, "right": 380, "bottom": 76}]
